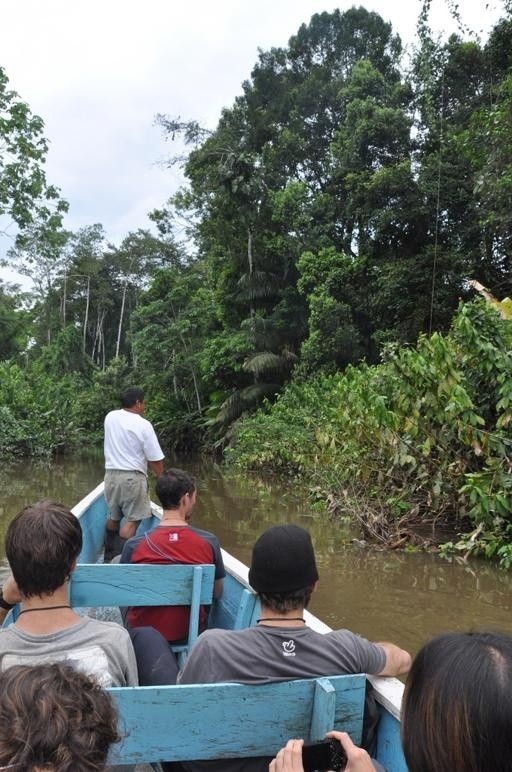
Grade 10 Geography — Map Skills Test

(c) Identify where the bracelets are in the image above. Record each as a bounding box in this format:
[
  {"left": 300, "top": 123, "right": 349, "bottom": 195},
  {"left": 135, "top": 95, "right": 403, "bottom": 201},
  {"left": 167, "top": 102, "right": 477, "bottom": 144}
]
[{"left": 0, "top": 588, "right": 16, "bottom": 609}]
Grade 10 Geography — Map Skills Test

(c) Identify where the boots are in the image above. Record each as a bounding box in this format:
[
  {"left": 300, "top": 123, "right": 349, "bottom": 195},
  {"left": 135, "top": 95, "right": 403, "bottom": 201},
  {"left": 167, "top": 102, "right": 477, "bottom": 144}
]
[
  {"left": 113, "top": 536, "right": 127, "bottom": 557},
  {"left": 104, "top": 527, "right": 118, "bottom": 562}
]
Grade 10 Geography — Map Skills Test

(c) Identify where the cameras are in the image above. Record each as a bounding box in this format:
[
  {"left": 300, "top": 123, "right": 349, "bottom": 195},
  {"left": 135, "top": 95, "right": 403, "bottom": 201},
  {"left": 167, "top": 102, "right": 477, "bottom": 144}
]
[{"left": 303, "top": 737, "right": 344, "bottom": 772}]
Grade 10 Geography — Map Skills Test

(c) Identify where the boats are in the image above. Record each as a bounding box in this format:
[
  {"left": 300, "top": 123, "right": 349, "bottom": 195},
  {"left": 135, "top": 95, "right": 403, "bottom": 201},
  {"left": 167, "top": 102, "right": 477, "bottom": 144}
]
[{"left": 0, "top": 481, "right": 406, "bottom": 772}]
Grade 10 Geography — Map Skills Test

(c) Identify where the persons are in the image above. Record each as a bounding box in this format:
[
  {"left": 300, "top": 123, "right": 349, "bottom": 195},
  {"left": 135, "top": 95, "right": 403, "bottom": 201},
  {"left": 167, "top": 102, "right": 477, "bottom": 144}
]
[
  {"left": 128, "top": 526, "right": 413, "bottom": 771},
  {"left": 109, "top": 467, "right": 226, "bottom": 644},
  {"left": 0, "top": 499, "right": 163, "bottom": 772},
  {"left": 102, "top": 387, "right": 165, "bottom": 564},
  {"left": 0, "top": 658, "right": 120, "bottom": 772},
  {"left": 268, "top": 629, "right": 512, "bottom": 772}
]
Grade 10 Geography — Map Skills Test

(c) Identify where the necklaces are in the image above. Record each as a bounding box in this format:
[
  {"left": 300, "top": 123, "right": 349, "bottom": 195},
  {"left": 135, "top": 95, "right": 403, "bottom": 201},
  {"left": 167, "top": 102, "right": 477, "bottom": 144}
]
[
  {"left": 257, "top": 617, "right": 306, "bottom": 627},
  {"left": 162, "top": 518, "right": 186, "bottom": 522}
]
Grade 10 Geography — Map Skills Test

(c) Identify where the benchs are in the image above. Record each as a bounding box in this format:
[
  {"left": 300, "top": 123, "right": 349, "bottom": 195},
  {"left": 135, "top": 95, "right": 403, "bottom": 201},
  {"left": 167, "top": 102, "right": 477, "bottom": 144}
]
[
  {"left": 104, "top": 676, "right": 364, "bottom": 762},
  {"left": 70, "top": 565, "right": 216, "bottom": 668}
]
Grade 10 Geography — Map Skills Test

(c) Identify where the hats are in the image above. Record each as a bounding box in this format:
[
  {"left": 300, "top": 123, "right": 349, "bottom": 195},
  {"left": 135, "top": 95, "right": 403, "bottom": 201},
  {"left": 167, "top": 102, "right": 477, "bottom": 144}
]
[{"left": 249, "top": 526, "right": 318, "bottom": 594}]
